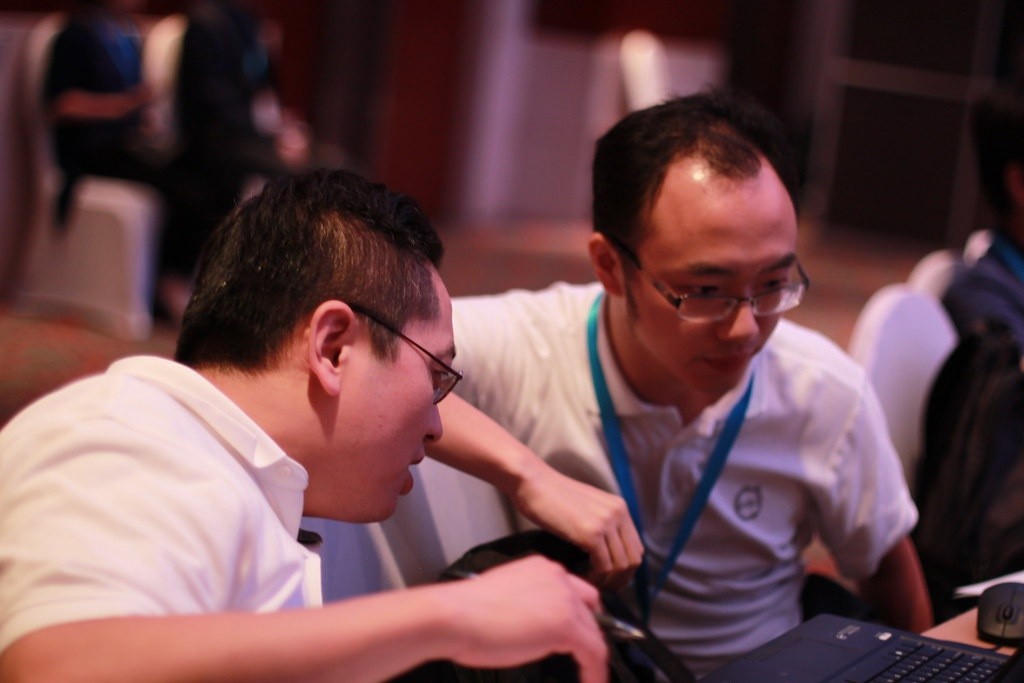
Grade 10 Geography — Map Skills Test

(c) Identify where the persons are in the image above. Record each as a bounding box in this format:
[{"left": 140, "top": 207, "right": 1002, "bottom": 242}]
[
  {"left": 424, "top": 92, "right": 931, "bottom": 683},
  {"left": 908, "top": 103, "right": 1024, "bottom": 627},
  {"left": 37, "top": 0, "right": 313, "bottom": 334},
  {"left": 0, "top": 171, "right": 611, "bottom": 683}
]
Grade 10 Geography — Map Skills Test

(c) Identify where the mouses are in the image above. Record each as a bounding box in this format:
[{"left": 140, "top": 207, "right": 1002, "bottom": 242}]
[{"left": 977, "top": 582, "right": 1024, "bottom": 646}]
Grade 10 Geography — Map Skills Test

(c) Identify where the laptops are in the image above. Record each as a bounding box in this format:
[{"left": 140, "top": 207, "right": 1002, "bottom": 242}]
[{"left": 693, "top": 613, "right": 1024, "bottom": 683}]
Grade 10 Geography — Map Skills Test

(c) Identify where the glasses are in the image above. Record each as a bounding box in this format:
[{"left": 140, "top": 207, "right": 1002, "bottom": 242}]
[
  {"left": 603, "top": 225, "right": 811, "bottom": 326},
  {"left": 345, "top": 300, "right": 463, "bottom": 405}
]
